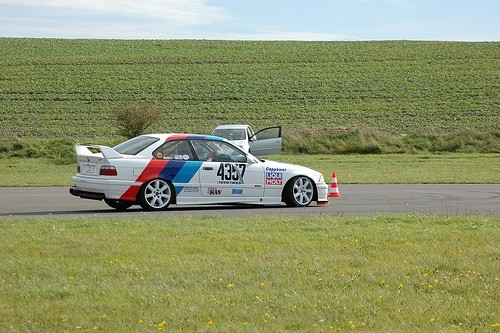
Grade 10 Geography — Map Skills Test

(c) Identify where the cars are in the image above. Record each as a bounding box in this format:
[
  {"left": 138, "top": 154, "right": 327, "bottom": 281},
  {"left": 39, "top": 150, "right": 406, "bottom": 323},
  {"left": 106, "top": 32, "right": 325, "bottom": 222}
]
[
  {"left": 212, "top": 124, "right": 282, "bottom": 157},
  {"left": 69, "top": 133, "right": 329, "bottom": 212}
]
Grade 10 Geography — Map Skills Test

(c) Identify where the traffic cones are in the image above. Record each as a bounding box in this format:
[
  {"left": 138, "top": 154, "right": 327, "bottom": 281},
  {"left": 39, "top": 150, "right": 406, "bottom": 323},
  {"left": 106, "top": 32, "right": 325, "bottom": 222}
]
[{"left": 328, "top": 172, "right": 341, "bottom": 198}]
